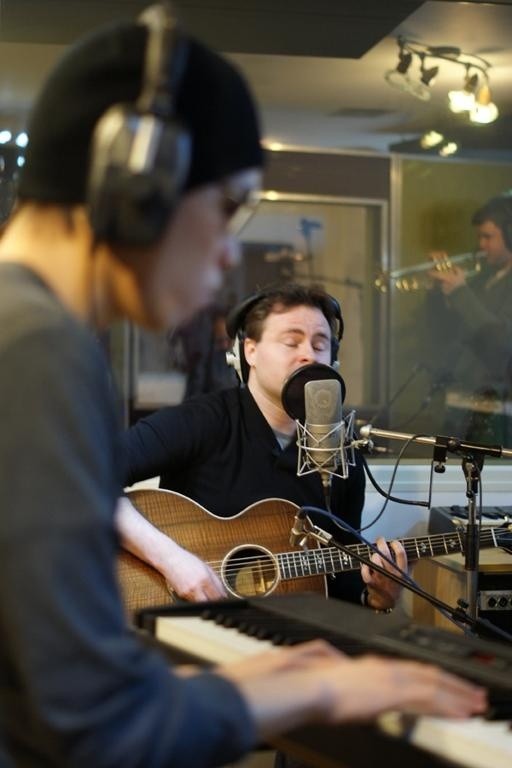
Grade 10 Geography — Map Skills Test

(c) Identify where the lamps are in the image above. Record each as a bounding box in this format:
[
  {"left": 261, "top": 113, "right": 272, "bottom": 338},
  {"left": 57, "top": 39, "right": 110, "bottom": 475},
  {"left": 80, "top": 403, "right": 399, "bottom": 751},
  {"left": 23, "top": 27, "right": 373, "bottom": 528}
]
[{"left": 386, "top": 34, "right": 498, "bottom": 123}]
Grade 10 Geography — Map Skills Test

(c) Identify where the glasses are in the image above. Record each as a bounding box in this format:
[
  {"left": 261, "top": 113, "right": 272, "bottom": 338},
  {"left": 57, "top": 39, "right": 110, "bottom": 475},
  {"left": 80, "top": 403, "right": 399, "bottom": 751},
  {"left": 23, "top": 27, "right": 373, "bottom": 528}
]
[{"left": 219, "top": 184, "right": 264, "bottom": 237}]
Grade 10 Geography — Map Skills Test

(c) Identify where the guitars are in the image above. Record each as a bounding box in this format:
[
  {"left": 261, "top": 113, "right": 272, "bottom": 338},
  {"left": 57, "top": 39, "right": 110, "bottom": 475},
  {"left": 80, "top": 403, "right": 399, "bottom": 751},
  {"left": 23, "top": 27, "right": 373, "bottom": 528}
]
[{"left": 110, "top": 487, "right": 512, "bottom": 629}]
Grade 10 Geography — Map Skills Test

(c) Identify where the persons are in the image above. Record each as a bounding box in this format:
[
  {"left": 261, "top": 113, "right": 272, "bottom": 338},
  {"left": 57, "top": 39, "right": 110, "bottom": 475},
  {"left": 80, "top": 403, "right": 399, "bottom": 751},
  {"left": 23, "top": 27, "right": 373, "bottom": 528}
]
[
  {"left": 422, "top": 194, "right": 512, "bottom": 457},
  {"left": 163, "top": 286, "right": 248, "bottom": 405},
  {"left": 2, "top": 24, "right": 494, "bottom": 767},
  {"left": 110, "top": 281, "right": 416, "bottom": 617}
]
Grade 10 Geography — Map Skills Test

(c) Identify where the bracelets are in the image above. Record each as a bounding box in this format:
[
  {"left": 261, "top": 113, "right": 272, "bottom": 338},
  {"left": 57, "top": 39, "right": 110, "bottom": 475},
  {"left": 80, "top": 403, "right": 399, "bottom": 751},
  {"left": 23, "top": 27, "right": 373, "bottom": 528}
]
[{"left": 363, "top": 588, "right": 394, "bottom": 615}]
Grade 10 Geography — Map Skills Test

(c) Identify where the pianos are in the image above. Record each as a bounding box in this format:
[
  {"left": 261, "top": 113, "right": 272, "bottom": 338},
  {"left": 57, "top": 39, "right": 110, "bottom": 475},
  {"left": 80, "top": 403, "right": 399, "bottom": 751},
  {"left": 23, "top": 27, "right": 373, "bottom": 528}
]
[{"left": 139, "top": 588, "right": 512, "bottom": 768}]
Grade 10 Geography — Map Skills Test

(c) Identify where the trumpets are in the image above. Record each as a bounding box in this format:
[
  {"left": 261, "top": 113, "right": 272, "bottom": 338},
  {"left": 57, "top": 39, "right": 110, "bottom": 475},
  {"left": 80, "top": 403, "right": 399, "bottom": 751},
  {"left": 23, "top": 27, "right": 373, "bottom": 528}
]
[{"left": 371, "top": 251, "right": 486, "bottom": 293}]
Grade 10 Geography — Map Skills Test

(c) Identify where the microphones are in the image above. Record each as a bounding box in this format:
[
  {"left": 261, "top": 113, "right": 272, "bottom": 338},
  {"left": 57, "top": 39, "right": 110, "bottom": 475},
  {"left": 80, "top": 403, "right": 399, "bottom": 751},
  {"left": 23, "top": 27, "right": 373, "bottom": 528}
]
[{"left": 302, "top": 377, "right": 345, "bottom": 495}]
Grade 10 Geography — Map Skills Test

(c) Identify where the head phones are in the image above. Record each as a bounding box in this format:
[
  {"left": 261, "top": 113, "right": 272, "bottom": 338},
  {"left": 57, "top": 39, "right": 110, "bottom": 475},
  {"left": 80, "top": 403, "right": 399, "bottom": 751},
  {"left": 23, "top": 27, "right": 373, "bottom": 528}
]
[
  {"left": 86, "top": 2, "right": 193, "bottom": 252},
  {"left": 225, "top": 284, "right": 345, "bottom": 384}
]
